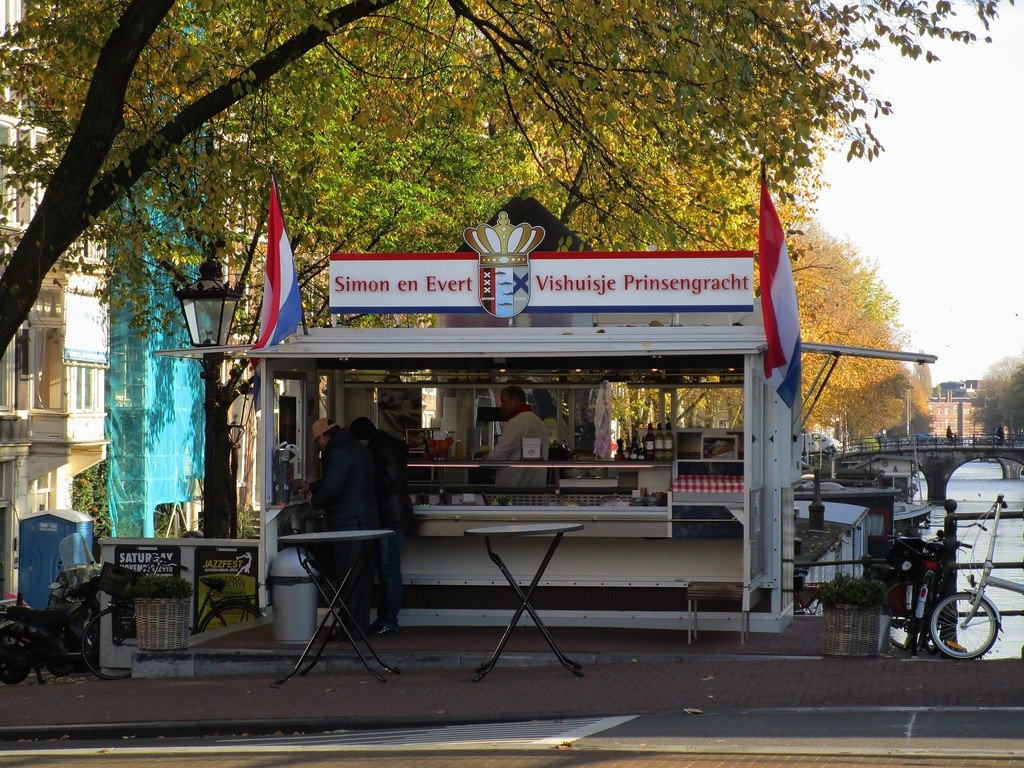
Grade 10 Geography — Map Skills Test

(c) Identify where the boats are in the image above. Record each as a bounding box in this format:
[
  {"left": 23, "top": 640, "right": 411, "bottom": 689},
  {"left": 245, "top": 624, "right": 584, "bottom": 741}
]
[{"left": 893, "top": 498, "right": 932, "bottom": 535}]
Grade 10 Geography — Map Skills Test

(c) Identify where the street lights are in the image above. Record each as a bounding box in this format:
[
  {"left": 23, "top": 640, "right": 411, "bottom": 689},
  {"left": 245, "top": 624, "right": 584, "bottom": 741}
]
[{"left": 176, "top": 255, "right": 242, "bottom": 539}]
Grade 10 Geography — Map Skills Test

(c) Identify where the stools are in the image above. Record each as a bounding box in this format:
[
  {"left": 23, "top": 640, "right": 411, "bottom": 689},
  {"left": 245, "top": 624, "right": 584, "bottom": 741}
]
[{"left": 685, "top": 578, "right": 747, "bottom": 645}]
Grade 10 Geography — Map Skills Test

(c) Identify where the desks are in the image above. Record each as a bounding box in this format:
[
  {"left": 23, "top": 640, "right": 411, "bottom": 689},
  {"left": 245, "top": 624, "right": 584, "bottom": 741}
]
[
  {"left": 273, "top": 530, "right": 401, "bottom": 686},
  {"left": 465, "top": 523, "right": 586, "bottom": 681}
]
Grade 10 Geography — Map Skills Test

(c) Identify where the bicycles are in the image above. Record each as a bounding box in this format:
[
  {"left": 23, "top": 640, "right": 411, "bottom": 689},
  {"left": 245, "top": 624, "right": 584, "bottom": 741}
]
[
  {"left": 930, "top": 494, "right": 1024, "bottom": 660},
  {"left": 81, "top": 553, "right": 264, "bottom": 681},
  {"left": 880, "top": 530, "right": 973, "bottom": 656}
]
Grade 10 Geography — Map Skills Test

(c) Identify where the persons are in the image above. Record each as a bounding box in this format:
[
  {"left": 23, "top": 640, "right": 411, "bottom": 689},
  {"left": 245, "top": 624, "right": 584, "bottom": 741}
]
[
  {"left": 877, "top": 432, "right": 883, "bottom": 449},
  {"left": 483, "top": 386, "right": 549, "bottom": 488},
  {"left": 347, "top": 418, "right": 408, "bottom": 636},
  {"left": 997, "top": 426, "right": 1005, "bottom": 443},
  {"left": 294, "top": 417, "right": 378, "bottom": 640},
  {"left": 946, "top": 426, "right": 953, "bottom": 443}
]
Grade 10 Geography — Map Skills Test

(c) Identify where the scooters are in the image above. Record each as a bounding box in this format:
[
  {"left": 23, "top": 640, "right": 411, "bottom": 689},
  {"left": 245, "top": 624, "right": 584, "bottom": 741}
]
[{"left": 0, "top": 532, "right": 101, "bottom": 685}]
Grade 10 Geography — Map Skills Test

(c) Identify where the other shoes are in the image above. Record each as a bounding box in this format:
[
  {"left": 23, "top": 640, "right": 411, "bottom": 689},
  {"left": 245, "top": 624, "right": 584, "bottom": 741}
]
[{"left": 327, "top": 632, "right": 368, "bottom": 642}]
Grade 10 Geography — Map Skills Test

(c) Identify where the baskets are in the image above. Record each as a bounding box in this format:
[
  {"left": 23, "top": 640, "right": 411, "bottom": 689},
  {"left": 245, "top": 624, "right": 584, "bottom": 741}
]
[
  {"left": 98, "top": 561, "right": 139, "bottom": 599},
  {"left": 822, "top": 604, "right": 881, "bottom": 658},
  {"left": 134, "top": 599, "right": 191, "bottom": 649}
]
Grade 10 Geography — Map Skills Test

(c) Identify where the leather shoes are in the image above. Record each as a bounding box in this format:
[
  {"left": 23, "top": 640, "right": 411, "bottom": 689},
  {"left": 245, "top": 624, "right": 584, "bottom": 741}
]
[{"left": 369, "top": 620, "right": 399, "bottom": 636}]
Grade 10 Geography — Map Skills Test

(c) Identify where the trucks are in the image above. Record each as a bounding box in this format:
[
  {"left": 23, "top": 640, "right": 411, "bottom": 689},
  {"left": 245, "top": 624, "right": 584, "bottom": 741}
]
[{"left": 801, "top": 432, "right": 839, "bottom": 455}]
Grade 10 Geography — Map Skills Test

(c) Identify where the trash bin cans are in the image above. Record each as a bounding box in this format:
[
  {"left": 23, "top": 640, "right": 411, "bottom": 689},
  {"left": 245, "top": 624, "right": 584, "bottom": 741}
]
[{"left": 268, "top": 547, "right": 318, "bottom": 644}]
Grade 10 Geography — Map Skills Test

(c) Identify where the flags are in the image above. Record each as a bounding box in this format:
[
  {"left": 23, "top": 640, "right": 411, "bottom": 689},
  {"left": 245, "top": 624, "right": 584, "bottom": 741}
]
[
  {"left": 251, "top": 174, "right": 302, "bottom": 412},
  {"left": 759, "top": 177, "right": 800, "bottom": 407}
]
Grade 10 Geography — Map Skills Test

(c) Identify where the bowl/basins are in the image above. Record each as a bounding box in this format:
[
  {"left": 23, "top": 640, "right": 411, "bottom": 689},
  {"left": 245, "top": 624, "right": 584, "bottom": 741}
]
[
  {"left": 630, "top": 497, "right": 645, "bottom": 506},
  {"left": 646, "top": 497, "right": 660, "bottom": 506}
]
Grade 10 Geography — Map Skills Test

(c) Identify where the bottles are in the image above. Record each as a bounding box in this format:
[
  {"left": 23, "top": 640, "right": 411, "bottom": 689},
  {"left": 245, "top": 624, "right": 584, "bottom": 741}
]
[
  {"left": 456, "top": 440, "right": 462, "bottom": 460},
  {"left": 628, "top": 422, "right": 673, "bottom": 461}
]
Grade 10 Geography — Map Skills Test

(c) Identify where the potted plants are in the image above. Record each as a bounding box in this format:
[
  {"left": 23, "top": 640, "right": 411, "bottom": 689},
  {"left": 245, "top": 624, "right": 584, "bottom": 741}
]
[
  {"left": 818, "top": 569, "right": 887, "bottom": 660},
  {"left": 132, "top": 574, "right": 193, "bottom": 650}
]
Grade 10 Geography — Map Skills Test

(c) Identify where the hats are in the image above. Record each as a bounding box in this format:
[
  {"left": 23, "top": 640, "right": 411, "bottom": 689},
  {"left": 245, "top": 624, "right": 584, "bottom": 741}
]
[
  {"left": 311, "top": 417, "right": 337, "bottom": 444},
  {"left": 348, "top": 416, "right": 377, "bottom": 440}
]
